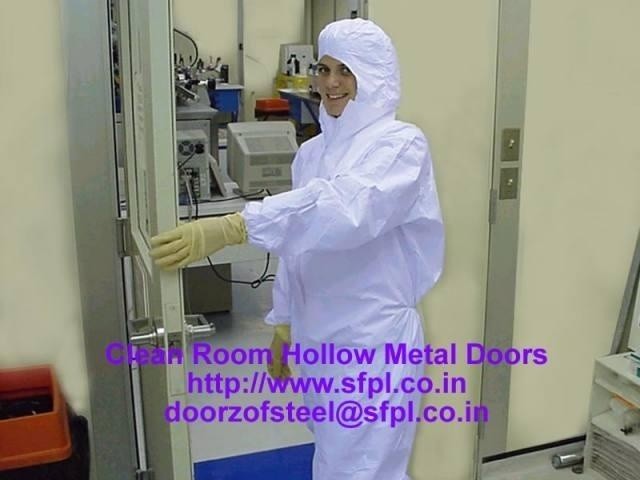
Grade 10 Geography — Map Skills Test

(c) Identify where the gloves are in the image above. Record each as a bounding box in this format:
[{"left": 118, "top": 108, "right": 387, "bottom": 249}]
[
  {"left": 266, "top": 325, "right": 292, "bottom": 382},
  {"left": 149, "top": 214, "right": 247, "bottom": 273}
]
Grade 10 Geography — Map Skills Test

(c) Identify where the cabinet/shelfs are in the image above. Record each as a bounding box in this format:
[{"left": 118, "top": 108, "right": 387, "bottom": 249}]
[{"left": 581, "top": 354, "right": 640, "bottom": 480}]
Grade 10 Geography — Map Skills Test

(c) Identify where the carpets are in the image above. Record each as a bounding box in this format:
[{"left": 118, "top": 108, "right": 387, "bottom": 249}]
[{"left": 192, "top": 443, "right": 314, "bottom": 480}]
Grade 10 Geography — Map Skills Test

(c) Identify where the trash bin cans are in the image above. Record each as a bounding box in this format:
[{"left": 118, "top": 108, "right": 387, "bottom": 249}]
[
  {"left": 254, "top": 97, "right": 290, "bottom": 120},
  {"left": 0, "top": 365, "right": 90, "bottom": 480}
]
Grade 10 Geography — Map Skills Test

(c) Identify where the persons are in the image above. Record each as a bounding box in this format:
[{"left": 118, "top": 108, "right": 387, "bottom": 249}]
[{"left": 144, "top": 18, "right": 448, "bottom": 480}]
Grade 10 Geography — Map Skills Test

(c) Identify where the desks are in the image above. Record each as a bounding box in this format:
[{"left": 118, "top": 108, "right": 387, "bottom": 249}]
[
  {"left": 213, "top": 83, "right": 244, "bottom": 123},
  {"left": 279, "top": 88, "right": 320, "bottom": 133}
]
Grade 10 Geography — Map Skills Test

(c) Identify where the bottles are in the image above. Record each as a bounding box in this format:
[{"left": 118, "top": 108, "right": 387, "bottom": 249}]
[
  {"left": 611, "top": 397, "right": 640, "bottom": 425},
  {"left": 287, "top": 54, "right": 300, "bottom": 76}
]
